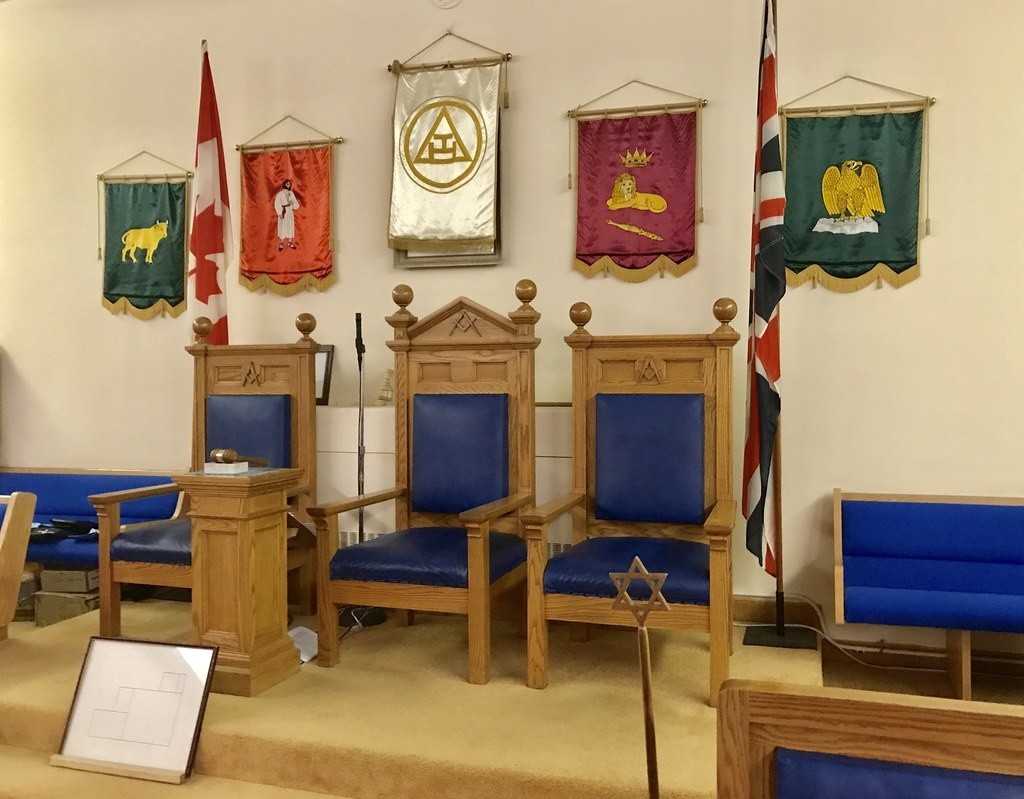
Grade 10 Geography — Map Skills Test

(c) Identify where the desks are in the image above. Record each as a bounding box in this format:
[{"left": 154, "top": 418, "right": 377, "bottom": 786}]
[{"left": 170, "top": 467, "right": 305, "bottom": 698}]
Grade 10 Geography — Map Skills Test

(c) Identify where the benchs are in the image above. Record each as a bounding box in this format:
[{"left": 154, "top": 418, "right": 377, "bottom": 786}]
[
  {"left": 1, "top": 467, "right": 191, "bottom": 565},
  {"left": 716, "top": 679, "right": 1023, "bottom": 799},
  {"left": 1, "top": 491, "right": 38, "bottom": 642},
  {"left": 832, "top": 486, "right": 1023, "bottom": 704}
]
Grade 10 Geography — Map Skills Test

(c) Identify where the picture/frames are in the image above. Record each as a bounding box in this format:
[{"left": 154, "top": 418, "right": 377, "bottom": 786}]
[{"left": 315, "top": 345, "right": 335, "bottom": 405}]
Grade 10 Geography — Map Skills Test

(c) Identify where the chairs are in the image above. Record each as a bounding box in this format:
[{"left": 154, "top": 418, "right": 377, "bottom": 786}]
[
  {"left": 305, "top": 280, "right": 541, "bottom": 685},
  {"left": 520, "top": 298, "right": 742, "bottom": 709},
  {"left": 87, "top": 313, "right": 319, "bottom": 639}
]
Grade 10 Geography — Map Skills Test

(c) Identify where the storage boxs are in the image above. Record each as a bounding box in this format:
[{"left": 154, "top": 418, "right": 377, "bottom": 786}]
[{"left": 13, "top": 561, "right": 100, "bottom": 626}]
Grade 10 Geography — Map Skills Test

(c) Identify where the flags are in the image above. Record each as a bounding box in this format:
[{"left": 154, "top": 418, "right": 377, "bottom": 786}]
[
  {"left": 186, "top": 40, "right": 234, "bottom": 345},
  {"left": 740, "top": 0, "right": 787, "bottom": 579}
]
